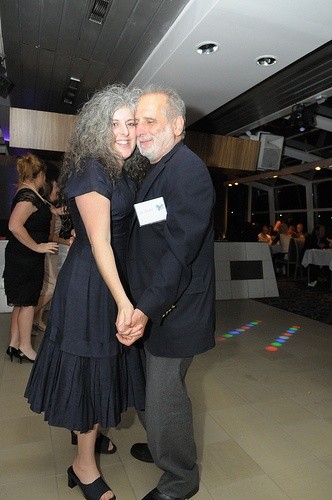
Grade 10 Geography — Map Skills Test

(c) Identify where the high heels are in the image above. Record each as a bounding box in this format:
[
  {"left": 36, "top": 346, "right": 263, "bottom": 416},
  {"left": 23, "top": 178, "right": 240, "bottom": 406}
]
[
  {"left": 7, "top": 346, "right": 19, "bottom": 362},
  {"left": 71, "top": 431, "right": 117, "bottom": 454},
  {"left": 17, "top": 349, "right": 35, "bottom": 364},
  {"left": 68, "top": 466, "right": 116, "bottom": 500}
]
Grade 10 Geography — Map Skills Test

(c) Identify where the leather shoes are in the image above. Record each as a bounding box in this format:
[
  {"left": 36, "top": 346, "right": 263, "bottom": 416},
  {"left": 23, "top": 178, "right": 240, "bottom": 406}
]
[
  {"left": 130, "top": 443, "right": 154, "bottom": 463},
  {"left": 141, "top": 485, "right": 199, "bottom": 500}
]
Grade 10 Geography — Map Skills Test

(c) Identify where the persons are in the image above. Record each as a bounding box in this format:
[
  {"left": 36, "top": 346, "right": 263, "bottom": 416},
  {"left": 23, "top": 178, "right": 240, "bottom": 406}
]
[
  {"left": 2, "top": 82, "right": 217, "bottom": 500},
  {"left": 258, "top": 221, "right": 332, "bottom": 289}
]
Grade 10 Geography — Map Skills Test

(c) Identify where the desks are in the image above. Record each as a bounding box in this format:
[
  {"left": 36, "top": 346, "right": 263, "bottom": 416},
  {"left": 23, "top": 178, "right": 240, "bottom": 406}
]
[{"left": 301, "top": 248, "right": 332, "bottom": 287}]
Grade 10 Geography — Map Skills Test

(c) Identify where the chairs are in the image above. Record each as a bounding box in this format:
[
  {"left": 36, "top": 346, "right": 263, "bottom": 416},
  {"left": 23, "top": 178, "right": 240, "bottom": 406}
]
[{"left": 275, "top": 238, "right": 305, "bottom": 281}]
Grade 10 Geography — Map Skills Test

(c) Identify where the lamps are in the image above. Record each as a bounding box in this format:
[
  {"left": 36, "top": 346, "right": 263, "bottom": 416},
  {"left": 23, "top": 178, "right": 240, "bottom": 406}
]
[{"left": 288, "top": 103, "right": 315, "bottom": 132}]
